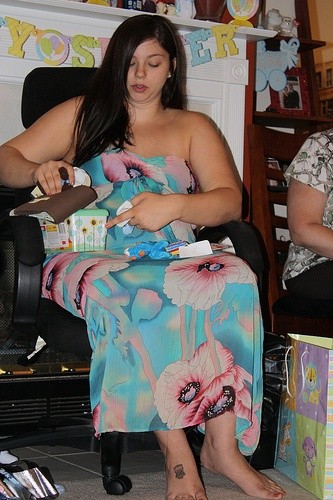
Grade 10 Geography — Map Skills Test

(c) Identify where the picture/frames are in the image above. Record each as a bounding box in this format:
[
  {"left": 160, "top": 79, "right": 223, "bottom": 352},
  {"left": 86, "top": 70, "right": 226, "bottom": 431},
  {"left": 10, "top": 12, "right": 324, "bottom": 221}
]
[{"left": 269, "top": 67, "right": 312, "bottom": 118}]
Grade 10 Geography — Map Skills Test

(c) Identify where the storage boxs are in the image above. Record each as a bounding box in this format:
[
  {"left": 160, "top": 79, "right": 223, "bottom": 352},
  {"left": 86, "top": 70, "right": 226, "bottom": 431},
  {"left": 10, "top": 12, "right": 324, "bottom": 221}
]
[{"left": 39, "top": 209, "right": 110, "bottom": 254}]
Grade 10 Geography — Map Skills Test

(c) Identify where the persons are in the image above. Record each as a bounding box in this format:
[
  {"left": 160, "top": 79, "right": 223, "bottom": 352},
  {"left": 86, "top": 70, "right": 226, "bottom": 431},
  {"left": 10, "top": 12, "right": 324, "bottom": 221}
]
[
  {"left": 282, "top": 84, "right": 300, "bottom": 109},
  {"left": 0, "top": 14, "right": 287, "bottom": 500},
  {"left": 283, "top": 128, "right": 333, "bottom": 318}
]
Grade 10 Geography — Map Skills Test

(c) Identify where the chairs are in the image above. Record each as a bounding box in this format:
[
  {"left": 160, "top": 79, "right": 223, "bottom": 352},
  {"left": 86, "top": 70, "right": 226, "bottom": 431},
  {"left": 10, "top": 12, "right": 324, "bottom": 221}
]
[
  {"left": 0, "top": 67, "right": 274, "bottom": 495},
  {"left": 247, "top": 124, "right": 333, "bottom": 338}
]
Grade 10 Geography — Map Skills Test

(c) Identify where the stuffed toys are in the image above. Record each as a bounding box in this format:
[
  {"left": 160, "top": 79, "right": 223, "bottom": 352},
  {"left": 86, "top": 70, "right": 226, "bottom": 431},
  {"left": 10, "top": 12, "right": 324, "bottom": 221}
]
[{"left": 262, "top": 8, "right": 298, "bottom": 38}]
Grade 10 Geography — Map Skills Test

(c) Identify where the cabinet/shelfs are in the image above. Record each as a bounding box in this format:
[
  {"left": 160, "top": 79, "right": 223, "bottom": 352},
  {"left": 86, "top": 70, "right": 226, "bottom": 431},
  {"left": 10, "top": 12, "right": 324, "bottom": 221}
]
[{"left": 0, "top": 0, "right": 278, "bottom": 183}]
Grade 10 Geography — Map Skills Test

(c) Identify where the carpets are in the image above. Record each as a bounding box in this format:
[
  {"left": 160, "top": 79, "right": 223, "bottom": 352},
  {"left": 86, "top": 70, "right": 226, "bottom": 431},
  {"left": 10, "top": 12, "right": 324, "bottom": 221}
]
[{"left": 54, "top": 468, "right": 321, "bottom": 500}]
